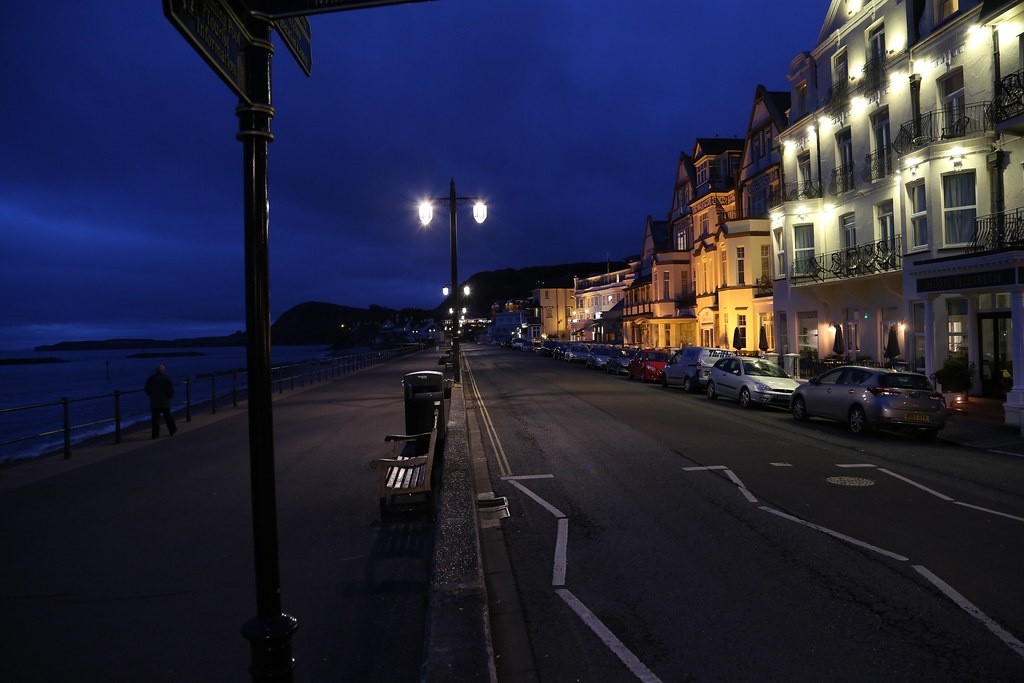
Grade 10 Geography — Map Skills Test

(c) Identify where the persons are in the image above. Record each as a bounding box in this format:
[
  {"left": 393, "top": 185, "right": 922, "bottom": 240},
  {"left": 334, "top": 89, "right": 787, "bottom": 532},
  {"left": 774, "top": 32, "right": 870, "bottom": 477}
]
[{"left": 144, "top": 364, "right": 178, "bottom": 439}]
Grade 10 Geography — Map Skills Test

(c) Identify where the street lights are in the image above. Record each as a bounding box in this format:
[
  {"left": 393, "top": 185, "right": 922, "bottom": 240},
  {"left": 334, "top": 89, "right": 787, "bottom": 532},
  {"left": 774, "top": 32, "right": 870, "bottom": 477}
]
[{"left": 415, "top": 176, "right": 489, "bottom": 385}]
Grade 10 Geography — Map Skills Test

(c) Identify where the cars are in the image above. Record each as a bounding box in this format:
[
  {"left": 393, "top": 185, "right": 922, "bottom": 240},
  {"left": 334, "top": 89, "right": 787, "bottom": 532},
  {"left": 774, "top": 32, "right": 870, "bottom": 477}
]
[
  {"left": 789, "top": 364, "right": 947, "bottom": 440},
  {"left": 509, "top": 337, "right": 590, "bottom": 363},
  {"left": 705, "top": 356, "right": 802, "bottom": 410},
  {"left": 607, "top": 347, "right": 638, "bottom": 376},
  {"left": 628, "top": 348, "right": 672, "bottom": 383},
  {"left": 585, "top": 347, "right": 612, "bottom": 369}
]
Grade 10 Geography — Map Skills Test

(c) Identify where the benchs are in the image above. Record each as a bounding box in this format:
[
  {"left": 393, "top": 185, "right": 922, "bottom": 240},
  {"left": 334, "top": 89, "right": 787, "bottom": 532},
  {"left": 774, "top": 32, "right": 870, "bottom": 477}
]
[
  {"left": 428, "top": 359, "right": 455, "bottom": 398},
  {"left": 374, "top": 409, "right": 439, "bottom": 525}
]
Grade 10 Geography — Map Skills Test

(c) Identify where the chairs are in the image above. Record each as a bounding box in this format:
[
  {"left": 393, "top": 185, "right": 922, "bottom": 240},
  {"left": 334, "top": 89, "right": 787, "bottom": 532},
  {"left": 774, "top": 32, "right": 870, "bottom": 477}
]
[{"left": 941, "top": 117, "right": 969, "bottom": 140}]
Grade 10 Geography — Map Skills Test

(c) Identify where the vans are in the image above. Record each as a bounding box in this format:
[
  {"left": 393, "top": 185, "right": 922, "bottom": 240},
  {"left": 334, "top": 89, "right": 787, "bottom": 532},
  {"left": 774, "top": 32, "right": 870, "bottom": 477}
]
[{"left": 661, "top": 346, "right": 733, "bottom": 393}]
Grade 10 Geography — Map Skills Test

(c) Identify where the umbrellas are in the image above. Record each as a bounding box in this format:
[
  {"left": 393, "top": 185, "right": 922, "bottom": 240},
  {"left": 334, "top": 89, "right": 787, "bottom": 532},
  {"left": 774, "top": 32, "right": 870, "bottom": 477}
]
[
  {"left": 884, "top": 325, "right": 901, "bottom": 365},
  {"left": 759, "top": 326, "right": 769, "bottom": 351},
  {"left": 733, "top": 327, "right": 743, "bottom": 350},
  {"left": 833, "top": 325, "right": 845, "bottom": 354}
]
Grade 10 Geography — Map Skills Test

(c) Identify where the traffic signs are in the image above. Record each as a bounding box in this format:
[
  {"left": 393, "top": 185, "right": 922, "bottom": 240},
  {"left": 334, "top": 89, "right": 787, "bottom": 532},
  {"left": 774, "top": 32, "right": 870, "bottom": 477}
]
[{"left": 164, "top": 0, "right": 315, "bottom": 109}]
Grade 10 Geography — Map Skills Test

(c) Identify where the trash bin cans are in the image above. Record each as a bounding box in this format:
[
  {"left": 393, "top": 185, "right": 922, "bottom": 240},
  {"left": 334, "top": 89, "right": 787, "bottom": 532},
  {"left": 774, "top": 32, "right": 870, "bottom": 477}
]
[
  {"left": 445, "top": 363, "right": 454, "bottom": 379},
  {"left": 404, "top": 369, "right": 446, "bottom": 448},
  {"left": 445, "top": 349, "right": 454, "bottom": 362}
]
